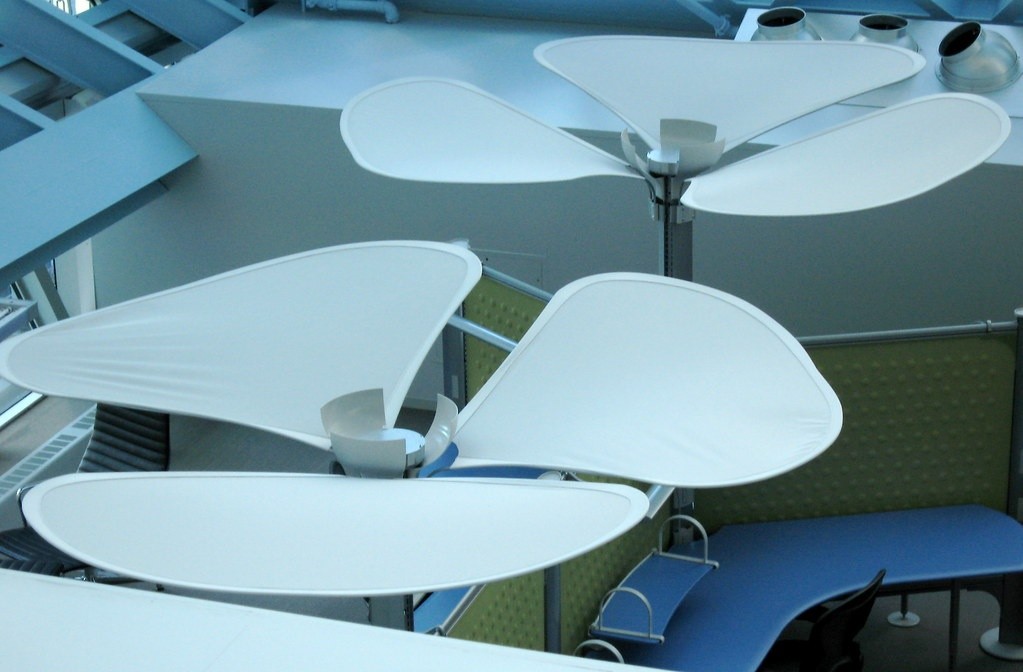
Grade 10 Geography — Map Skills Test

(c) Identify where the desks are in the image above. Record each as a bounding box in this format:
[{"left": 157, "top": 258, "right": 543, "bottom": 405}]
[{"left": 577, "top": 503, "right": 1023, "bottom": 672}]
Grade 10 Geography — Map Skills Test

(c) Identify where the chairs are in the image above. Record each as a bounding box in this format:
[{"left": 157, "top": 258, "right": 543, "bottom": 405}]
[{"left": 756, "top": 567, "right": 886, "bottom": 672}]
